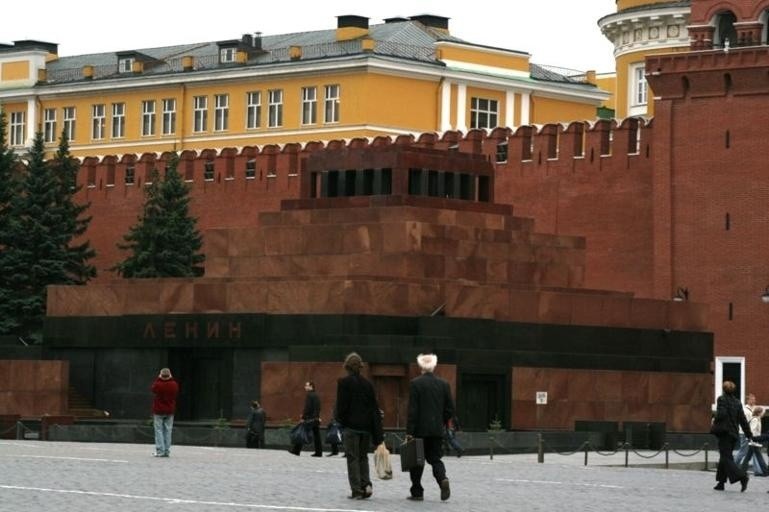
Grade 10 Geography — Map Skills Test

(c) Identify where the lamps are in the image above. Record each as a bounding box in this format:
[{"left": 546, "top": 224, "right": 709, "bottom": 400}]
[{"left": 673, "top": 287, "right": 689, "bottom": 304}]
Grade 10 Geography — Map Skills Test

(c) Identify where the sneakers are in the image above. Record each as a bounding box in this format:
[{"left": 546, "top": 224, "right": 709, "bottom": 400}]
[
  {"left": 288, "top": 449, "right": 339, "bottom": 457},
  {"left": 441, "top": 478, "right": 450, "bottom": 500},
  {"left": 714, "top": 476, "right": 748, "bottom": 492},
  {"left": 407, "top": 492, "right": 424, "bottom": 501},
  {"left": 348, "top": 487, "right": 373, "bottom": 500}
]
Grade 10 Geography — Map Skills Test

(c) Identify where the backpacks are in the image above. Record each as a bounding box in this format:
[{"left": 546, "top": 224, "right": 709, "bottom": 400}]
[{"left": 453, "top": 416, "right": 464, "bottom": 432}]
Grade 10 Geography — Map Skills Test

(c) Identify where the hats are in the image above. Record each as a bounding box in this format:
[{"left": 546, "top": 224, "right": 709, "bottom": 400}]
[{"left": 158, "top": 368, "right": 172, "bottom": 381}]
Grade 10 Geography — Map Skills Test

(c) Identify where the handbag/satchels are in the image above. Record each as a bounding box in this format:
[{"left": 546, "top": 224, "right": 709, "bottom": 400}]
[
  {"left": 324, "top": 423, "right": 343, "bottom": 444},
  {"left": 361, "top": 424, "right": 384, "bottom": 452},
  {"left": 374, "top": 443, "right": 392, "bottom": 480},
  {"left": 305, "top": 417, "right": 320, "bottom": 432},
  {"left": 289, "top": 422, "right": 308, "bottom": 444},
  {"left": 710, "top": 413, "right": 730, "bottom": 435}
]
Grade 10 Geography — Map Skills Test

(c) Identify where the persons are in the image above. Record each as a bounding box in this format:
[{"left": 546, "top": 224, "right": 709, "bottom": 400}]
[
  {"left": 148, "top": 367, "right": 179, "bottom": 458},
  {"left": 325, "top": 422, "right": 347, "bottom": 457},
  {"left": 245, "top": 400, "right": 267, "bottom": 449},
  {"left": 333, "top": 352, "right": 385, "bottom": 500},
  {"left": 405, "top": 353, "right": 453, "bottom": 501},
  {"left": 287, "top": 380, "right": 322, "bottom": 457},
  {"left": 710, "top": 380, "right": 769, "bottom": 493}
]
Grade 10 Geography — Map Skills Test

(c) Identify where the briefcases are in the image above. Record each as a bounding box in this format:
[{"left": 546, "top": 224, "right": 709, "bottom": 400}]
[{"left": 400, "top": 437, "right": 424, "bottom": 471}]
